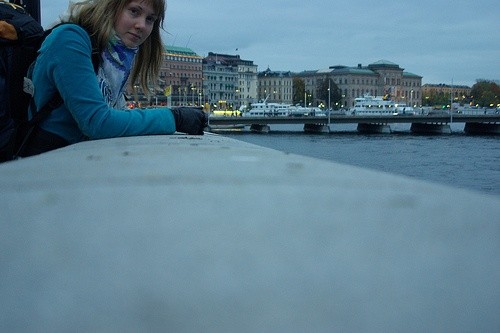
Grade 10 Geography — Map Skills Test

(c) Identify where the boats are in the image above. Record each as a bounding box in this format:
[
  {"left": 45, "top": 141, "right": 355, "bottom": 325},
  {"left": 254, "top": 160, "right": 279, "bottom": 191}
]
[
  {"left": 213, "top": 108, "right": 242, "bottom": 116},
  {"left": 246, "top": 103, "right": 327, "bottom": 116},
  {"left": 348, "top": 97, "right": 413, "bottom": 116}
]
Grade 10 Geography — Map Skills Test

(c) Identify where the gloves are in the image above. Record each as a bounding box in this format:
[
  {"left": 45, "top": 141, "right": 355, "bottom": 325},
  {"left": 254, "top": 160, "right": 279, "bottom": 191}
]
[{"left": 170, "top": 107, "right": 208, "bottom": 135}]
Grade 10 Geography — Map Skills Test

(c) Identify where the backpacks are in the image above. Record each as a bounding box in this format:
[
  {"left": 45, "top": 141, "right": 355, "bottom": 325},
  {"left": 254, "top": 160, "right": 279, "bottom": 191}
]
[{"left": 0, "top": 0, "right": 104, "bottom": 164}]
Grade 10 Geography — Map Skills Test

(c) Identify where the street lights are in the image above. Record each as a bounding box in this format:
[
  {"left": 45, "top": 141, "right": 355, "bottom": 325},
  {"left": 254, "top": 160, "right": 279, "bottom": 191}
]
[
  {"left": 308, "top": 95, "right": 312, "bottom": 104},
  {"left": 273, "top": 90, "right": 278, "bottom": 94},
  {"left": 318, "top": 102, "right": 325, "bottom": 108},
  {"left": 235, "top": 89, "right": 240, "bottom": 93},
  {"left": 328, "top": 89, "right": 331, "bottom": 124},
  {"left": 410, "top": 89, "right": 414, "bottom": 106}
]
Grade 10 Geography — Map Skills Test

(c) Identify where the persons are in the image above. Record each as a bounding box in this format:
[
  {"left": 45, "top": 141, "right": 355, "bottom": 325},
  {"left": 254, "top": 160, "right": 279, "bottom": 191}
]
[{"left": 15, "top": 0, "right": 208, "bottom": 161}]
